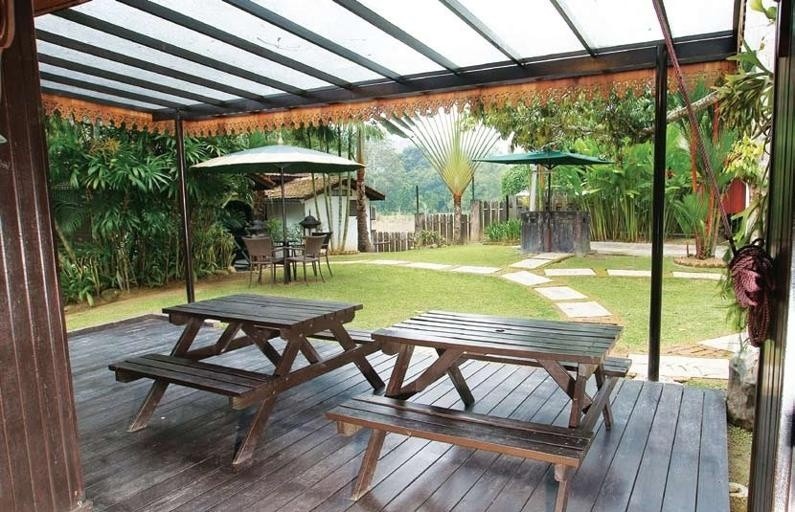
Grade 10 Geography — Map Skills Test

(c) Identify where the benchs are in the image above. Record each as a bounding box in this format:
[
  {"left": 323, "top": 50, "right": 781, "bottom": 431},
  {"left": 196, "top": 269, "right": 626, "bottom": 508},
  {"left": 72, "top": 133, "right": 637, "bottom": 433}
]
[
  {"left": 323, "top": 392, "right": 597, "bottom": 470},
  {"left": 109, "top": 350, "right": 272, "bottom": 415},
  {"left": 455, "top": 352, "right": 633, "bottom": 384},
  {"left": 252, "top": 321, "right": 379, "bottom": 353}
]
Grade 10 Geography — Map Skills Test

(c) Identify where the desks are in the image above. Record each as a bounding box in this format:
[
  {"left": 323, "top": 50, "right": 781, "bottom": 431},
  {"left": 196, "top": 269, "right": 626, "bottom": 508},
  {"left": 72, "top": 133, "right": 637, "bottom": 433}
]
[
  {"left": 348, "top": 306, "right": 625, "bottom": 511},
  {"left": 128, "top": 291, "right": 386, "bottom": 468}
]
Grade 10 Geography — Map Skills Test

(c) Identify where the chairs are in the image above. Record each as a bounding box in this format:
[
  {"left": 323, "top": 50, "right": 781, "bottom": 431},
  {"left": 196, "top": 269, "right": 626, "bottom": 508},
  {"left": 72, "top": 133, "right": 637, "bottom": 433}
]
[{"left": 241, "top": 231, "right": 333, "bottom": 289}]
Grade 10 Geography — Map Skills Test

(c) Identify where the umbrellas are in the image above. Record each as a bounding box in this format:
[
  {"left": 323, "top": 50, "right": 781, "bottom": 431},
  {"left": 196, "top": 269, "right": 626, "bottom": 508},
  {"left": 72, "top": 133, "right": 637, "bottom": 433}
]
[
  {"left": 190, "top": 141, "right": 367, "bottom": 283},
  {"left": 473, "top": 144, "right": 617, "bottom": 253}
]
